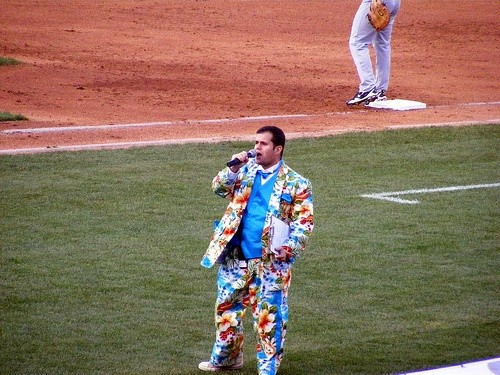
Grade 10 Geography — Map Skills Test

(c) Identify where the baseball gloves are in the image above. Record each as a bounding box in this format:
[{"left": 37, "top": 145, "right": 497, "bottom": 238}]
[{"left": 366, "top": 0, "right": 391, "bottom": 31}]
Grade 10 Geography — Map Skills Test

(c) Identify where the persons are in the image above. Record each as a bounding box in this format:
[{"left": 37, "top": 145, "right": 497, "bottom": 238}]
[
  {"left": 346, "top": 0, "right": 401, "bottom": 106},
  {"left": 198, "top": 126, "right": 314, "bottom": 375}
]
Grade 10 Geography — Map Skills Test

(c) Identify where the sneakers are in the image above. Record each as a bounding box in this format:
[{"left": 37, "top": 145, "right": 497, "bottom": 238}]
[
  {"left": 198, "top": 362, "right": 243, "bottom": 371},
  {"left": 365, "top": 91, "right": 386, "bottom": 105},
  {"left": 346, "top": 87, "right": 377, "bottom": 105}
]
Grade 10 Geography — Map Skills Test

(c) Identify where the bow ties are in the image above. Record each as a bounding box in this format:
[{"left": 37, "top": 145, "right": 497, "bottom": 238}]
[{"left": 257, "top": 165, "right": 280, "bottom": 179}]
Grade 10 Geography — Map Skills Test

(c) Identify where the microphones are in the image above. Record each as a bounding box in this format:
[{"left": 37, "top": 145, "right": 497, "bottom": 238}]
[{"left": 227, "top": 149, "right": 257, "bottom": 167}]
